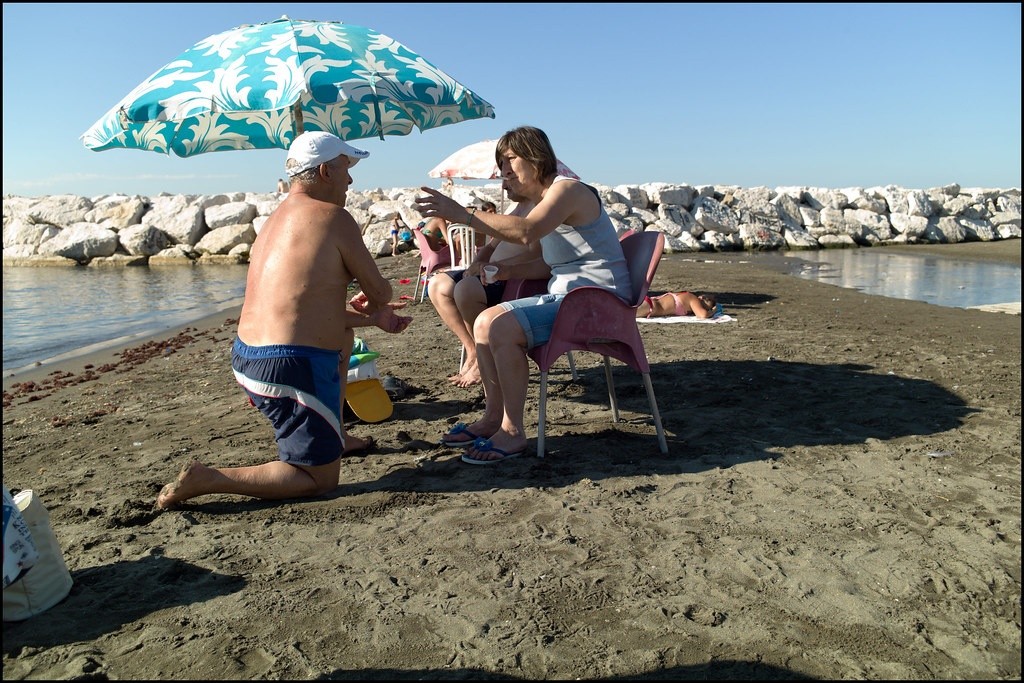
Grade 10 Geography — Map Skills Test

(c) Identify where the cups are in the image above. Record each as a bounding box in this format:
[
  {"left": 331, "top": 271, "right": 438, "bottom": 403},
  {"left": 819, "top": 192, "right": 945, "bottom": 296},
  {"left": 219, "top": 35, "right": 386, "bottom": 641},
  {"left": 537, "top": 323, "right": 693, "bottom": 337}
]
[{"left": 483, "top": 265, "right": 498, "bottom": 283}]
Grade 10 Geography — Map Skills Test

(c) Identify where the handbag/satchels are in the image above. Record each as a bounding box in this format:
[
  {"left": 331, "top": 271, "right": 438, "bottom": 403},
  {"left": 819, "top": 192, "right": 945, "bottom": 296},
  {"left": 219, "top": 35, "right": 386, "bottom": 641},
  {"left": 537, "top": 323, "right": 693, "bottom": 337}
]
[{"left": 1, "top": 482, "right": 73, "bottom": 621}]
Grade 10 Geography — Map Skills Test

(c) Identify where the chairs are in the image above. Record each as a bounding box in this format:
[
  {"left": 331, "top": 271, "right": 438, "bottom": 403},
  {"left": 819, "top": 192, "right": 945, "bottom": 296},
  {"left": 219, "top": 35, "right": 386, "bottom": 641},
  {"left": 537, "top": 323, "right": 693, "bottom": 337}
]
[
  {"left": 411, "top": 223, "right": 488, "bottom": 305},
  {"left": 502, "top": 228, "right": 669, "bottom": 457}
]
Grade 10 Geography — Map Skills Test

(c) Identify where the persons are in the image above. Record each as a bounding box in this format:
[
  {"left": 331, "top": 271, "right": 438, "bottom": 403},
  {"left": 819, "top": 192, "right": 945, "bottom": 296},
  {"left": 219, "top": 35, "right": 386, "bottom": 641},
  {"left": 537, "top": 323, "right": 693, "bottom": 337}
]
[
  {"left": 418, "top": 217, "right": 431, "bottom": 230},
  {"left": 636, "top": 291, "right": 718, "bottom": 319},
  {"left": 391, "top": 213, "right": 405, "bottom": 256},
  {"left": 158, "top": 130, "right": 414, "bottom": 514},
  {"left": 426, "top": 201, "right": 551, "bottom": 390},
  {"left": 481, "top": 201, "right": 497, "bottom": 215},
  {"left": 421, "top": 219, "right": 448, "bottom": 252},
  {"left": 411, "top": 124, "right": 632, "bottom": 464}
]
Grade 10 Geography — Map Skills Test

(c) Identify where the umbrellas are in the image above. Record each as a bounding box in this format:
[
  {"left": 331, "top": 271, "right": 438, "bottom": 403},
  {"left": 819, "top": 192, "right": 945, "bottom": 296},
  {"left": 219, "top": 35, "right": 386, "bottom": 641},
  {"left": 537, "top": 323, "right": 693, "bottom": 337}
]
[
  {"left": 428, "top": 139, "right": 579, "bottom": 214},
  {"left": 81, "top": 12, "right": 498, "bottom": 157}
]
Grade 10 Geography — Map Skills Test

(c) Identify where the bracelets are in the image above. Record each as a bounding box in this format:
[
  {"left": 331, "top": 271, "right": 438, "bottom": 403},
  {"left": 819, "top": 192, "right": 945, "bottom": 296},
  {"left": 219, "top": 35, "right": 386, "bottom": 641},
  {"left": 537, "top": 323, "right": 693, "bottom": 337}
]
[{"left": 468, "top": 208, "right": 475, "bottom": 227}]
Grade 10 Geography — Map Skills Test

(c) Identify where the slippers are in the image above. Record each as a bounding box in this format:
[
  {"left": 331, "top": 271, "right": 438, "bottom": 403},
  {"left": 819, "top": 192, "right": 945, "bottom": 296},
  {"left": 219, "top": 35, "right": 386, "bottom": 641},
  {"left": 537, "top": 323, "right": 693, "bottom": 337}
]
[
  {"left": 443, "top": 423, "right": 479, "bottom": 446},
  {"left": 462, "top": 436, "right": 522, "bottom": 463}
]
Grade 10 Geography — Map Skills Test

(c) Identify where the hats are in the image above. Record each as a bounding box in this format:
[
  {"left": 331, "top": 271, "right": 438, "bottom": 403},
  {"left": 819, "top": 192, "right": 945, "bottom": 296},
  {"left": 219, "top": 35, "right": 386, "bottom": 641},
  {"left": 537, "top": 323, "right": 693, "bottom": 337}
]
[{"left": 286, "top": 131, "right": 370, "bottom": 177}]
